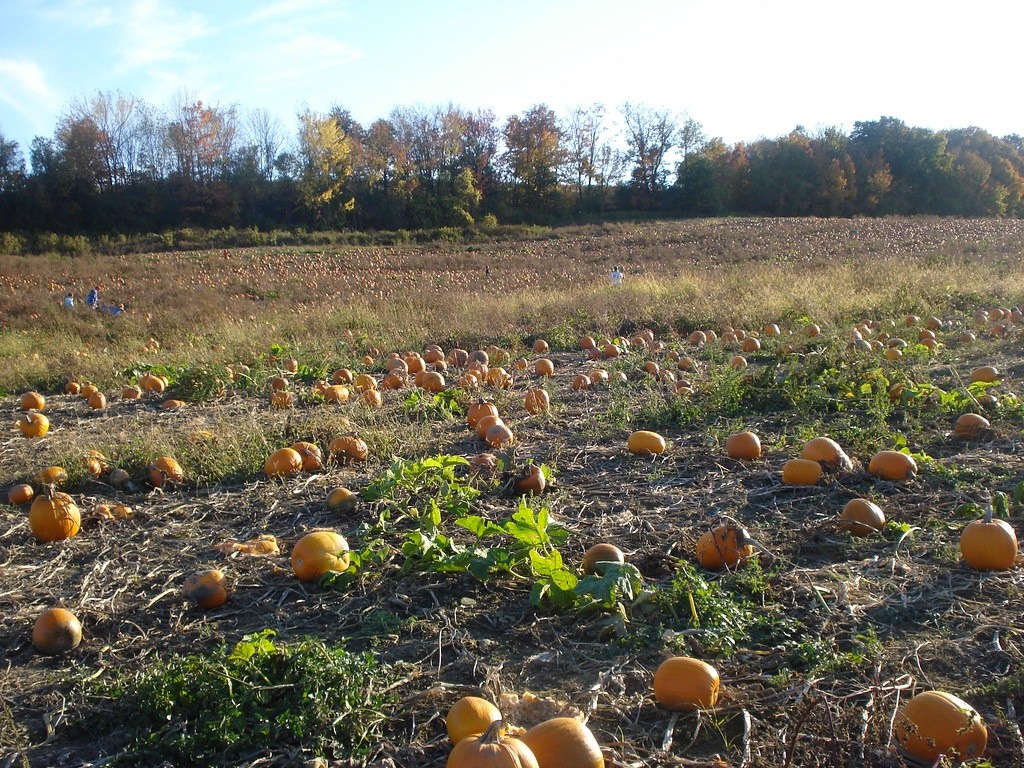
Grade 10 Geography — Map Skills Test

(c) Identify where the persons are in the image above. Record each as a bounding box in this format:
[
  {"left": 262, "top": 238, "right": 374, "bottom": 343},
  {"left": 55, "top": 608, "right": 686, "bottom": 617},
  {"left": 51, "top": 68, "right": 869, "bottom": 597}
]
[
  {"left": 85, "top": 284, "right": 102, "bottom": 310},
  {"left": 64, "top": 292, "right": 74, "bottom": 309},
  {"left": 608, "top": 265, "right": 623, "bottom": 286},
  {"left": 103, "top": 299, "right": 126, "bottom": 317},
  {"left": 485, "top": 265, "right": 492, "bottom": 276}
]
